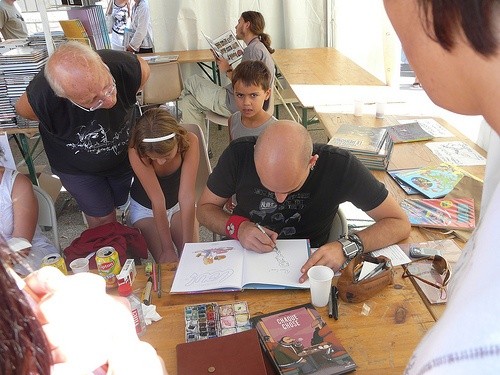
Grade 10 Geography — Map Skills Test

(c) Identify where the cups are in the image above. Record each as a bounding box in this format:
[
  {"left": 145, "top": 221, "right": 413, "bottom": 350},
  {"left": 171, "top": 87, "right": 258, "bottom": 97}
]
[
  {"left": 70, "top": 258, "right": 89, "bottom": 274},
  {"left": 375, "top": 101, "right": 386, "bottom": 118},
  {"left": 307, "top": 266, "right": 334, "bottom": 307},
  {"left": 353, "top": 99, "right": 363, "bottom": 116}
]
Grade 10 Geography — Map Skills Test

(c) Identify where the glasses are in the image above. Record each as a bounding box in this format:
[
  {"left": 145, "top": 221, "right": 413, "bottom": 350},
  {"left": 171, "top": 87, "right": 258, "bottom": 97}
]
[
  {"left": 65, "top": 71, "right": 116, "bottom": 111},
  {"left": 401, "top": 253, "right": 452, "bottom": 292}
]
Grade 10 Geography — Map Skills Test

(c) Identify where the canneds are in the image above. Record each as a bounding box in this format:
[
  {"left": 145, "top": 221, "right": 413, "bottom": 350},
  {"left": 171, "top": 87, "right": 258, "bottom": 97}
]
[
  {"left": 95, "top": 246, "right": 121, "bottom": 289},
  {"left": 40, "top": 253, "right": 68, "bottom": 276}
]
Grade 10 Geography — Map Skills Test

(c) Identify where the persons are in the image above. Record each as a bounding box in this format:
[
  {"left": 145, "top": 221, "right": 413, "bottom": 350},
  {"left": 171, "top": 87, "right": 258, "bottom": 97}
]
[
  {"left": 126, "top": 0, "right": 155, "bottom": 53},
  {"left": 383, "top": 0, "right": 500, "bottom": 375},
  {"left": 179, "top": 10, "right": 277, "bottom": 158},
  {"left": 224, "top": 61, "right": 279, "bottom": 214},
  {"left": 0, "top": 165, "right": 60, "bottom": 277},
  {"left": 125, "top": 108, "right": 206, "bottom": 264},
  {"left": 105, "top": 0, "right": 134, "bottom": 51},
  {"left": 15, "top": 40, "right": 151, "bottom": 228},
  {"left": 0, "top": 0, "right": 28, "bottom": 42},
  {"left": 0, "top": 234, "right": 163, "bottom": 375},
  {"left": 195, "top": 121, "right": 411, "bottom": 284}
]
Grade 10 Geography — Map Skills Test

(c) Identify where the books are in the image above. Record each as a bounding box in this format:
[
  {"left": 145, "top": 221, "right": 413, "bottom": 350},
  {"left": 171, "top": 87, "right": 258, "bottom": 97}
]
[
  {"left": 200, "top": 30, "right": 245, "bottom": 66},
  {"left": 169, "top": 240, "right": 315, "bottom": 292},
  {"left": 174, "top": 303, "right": 358, "bottom": 375},
  {"left": 0, "top": 31, "right": 68, "bottom": 128},
  {"left": 60, "top": 4, "right": 112, "bottom": 52},
  {"left": 326, "top": 122, "right": 476, "bottom": 230}
]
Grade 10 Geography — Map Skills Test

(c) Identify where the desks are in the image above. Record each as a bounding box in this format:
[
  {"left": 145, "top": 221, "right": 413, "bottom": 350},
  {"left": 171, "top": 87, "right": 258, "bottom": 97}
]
[
  {"left": 271, "top": 45, "right": 386, "bottom": 131},
  {"left": 313, "top": 106, "right": 489, "bottom": 323},
  {"left": 136, "top": 45, "right": 224, "bottom": 131},
  {"left": 0, "top": 127, "right": 40, "bottom": 187},
  {"left": 23, "top": 257, "right": 434, "bottom": 375}
]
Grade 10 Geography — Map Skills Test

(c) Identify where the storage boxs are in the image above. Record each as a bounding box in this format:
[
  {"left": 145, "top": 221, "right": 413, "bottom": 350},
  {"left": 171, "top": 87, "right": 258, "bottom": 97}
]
[{"left": 15, "top": 162, "right": 66, "bottom": 232}]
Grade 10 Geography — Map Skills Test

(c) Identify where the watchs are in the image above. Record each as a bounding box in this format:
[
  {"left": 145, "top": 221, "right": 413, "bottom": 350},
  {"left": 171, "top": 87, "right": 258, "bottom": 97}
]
[{"left": 336, "top": 238, "right": 358, "bottom": 260}]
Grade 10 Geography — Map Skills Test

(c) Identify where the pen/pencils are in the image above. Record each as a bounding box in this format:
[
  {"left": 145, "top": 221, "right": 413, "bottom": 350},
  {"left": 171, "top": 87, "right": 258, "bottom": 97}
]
[
  {"left": 140, "top": 260, "right": 161, "bottom": 303},
  {"left": 325, "top": 284, "right": 341, "bottom": 321},
  {"left": 255, "top": 222, "right": 281, "bottom": 255},
  {"left": 353, "top": 260, "right": 387, "bottom": 283}
]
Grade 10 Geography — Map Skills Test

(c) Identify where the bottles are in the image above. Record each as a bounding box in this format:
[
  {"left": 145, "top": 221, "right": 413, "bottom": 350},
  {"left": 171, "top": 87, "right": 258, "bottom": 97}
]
[{"left": 118, "top": 285, "right": 146, "bottom": 338}]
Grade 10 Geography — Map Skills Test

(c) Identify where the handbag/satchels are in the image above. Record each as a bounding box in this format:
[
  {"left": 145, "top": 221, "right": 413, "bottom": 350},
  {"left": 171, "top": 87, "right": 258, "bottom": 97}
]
[{"left": 104, "top": 1, "right": 114, "bottom": 35}]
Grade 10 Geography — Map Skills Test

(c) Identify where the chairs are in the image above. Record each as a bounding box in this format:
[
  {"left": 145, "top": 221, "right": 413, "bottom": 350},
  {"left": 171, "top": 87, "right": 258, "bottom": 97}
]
[
  {"left": 137, "top": 61, "right": 185, "bottom": 123},
  {"left": 274, "top": 73, "right": 303, "bottom": 125},
  {"left": 205, "top": 68, "right": 275, "bottom": 153},
  {"left": 179, "top": 122, "right": 224, "bottom": 243},
  {"left": 31, "top": 184, "right": 61, "bottom": 254}
]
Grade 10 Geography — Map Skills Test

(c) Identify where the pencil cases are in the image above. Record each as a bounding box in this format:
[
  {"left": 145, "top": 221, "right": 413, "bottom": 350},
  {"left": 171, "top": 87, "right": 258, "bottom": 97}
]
[{"left": 337, "top": 253, "right": 394, "bottom": 304}]
